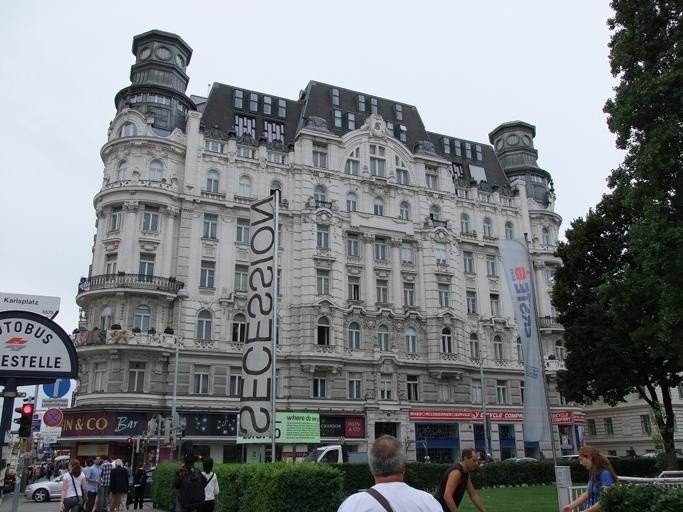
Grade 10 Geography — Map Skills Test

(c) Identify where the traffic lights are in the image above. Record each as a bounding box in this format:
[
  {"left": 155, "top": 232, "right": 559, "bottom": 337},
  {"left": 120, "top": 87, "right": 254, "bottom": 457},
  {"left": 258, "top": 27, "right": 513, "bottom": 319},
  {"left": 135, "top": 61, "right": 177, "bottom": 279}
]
[
  {"left": 19, "top": 403, "right": 34, "bottom": 438},
  {"left": 125, "top": 436, "right": 134, "bottom": 451},
  {"left": 12, "top": 406, "right": 20, "bottom": 435}
]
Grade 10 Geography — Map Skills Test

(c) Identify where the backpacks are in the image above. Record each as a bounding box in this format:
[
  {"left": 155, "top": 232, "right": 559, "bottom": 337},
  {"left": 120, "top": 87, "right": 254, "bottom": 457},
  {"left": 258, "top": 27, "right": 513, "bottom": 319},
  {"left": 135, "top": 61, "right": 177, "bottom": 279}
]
[{"left": 179, "top": 467, "right": 204, "bottom": 508}]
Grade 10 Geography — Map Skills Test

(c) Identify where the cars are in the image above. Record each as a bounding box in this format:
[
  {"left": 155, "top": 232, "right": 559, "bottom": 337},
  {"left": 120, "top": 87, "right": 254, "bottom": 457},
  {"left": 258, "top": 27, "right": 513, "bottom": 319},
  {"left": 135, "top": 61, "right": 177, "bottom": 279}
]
[
  {"left": 124, "top": 465, "right": 155, "bottom": 504},
  {"left": 500, "top": 451, "right": 682, "bottom": 464},
  {"left": 1, "top": 459, "right": 52, "bottom": 496},
  {"left": 23, "top": 470, "right": 71, "bottom": 503}
]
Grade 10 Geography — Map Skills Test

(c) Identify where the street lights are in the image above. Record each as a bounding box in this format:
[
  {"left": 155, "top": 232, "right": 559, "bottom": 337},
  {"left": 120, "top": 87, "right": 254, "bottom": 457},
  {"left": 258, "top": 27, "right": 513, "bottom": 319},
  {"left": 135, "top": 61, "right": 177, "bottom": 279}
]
[{"left": 168, "top": 284, "right": 191, "bottom": 459}]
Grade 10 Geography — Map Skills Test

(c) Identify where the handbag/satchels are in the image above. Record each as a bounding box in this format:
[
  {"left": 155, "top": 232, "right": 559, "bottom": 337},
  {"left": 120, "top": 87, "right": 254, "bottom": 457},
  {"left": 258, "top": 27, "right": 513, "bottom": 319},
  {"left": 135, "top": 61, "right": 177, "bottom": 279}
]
[
  {"left": 138, "top": 471, "right": 145, "bottom": 488},
  {"left": 79, "top": 504, "right": 83, "bottom": 512}
]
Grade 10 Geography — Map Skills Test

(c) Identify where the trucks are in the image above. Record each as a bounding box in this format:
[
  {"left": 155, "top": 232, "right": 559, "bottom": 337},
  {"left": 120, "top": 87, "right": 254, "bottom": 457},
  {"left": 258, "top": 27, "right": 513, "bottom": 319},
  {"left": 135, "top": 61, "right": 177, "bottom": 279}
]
[{"left": 301, "top": 440, "right": 430, "bottom": 463}]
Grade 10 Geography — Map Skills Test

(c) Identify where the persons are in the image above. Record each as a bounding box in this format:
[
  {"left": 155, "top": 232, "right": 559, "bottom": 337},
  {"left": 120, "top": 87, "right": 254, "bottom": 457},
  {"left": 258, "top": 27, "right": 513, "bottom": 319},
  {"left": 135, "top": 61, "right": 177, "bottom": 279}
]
[
  {"left": 174, "top": 446, "right": 207, "bottom": 512},
  {"left": 561, "top": 446, "right": 621, "bottom": 512},
  {"left": 337, "top": 434, "right": 444, "bottom": 512},
  {"left": 46, "top": 455, "right": 149, "bottom": 512},
  {"left": 201, "top": 457, "right": 219, "bottom": 512},
  {"left": 434, "top": 448, "right": 486, "bottom": 512}
]
